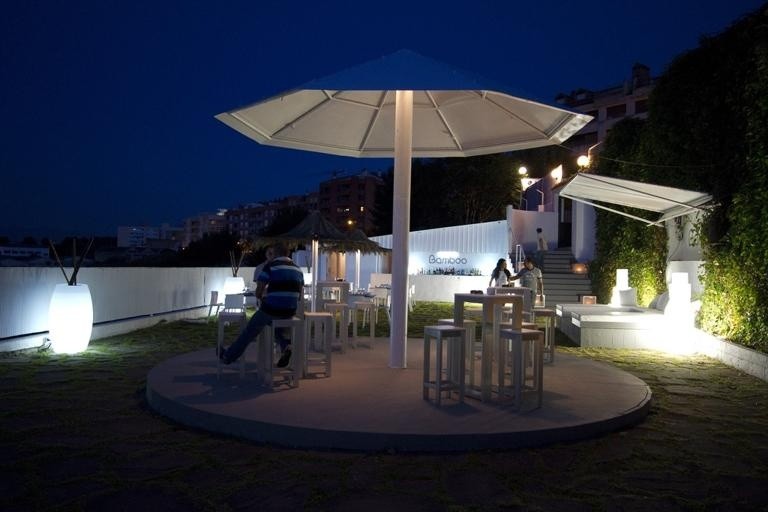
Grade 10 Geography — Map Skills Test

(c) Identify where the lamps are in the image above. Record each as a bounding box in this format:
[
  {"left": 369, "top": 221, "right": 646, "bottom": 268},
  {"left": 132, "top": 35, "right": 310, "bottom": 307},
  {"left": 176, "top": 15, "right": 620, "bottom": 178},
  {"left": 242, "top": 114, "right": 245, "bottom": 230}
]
[
  {"left": 48, "top": 236, "right": 94, "bottom": 357},
  {"left": 576, "top": 155, "right": 589, "bottom": 173},
  {"left": 551, "top": 169, "right": 560, "bottom": 181},
  {"left": 221, "top": 252, "right": 246, "bottom": 313},
  {"left": 302, "top": 256, "right": 313, "bottom": 286},
  {"left": 519, "top": 167, "right": 528, "bottom": 176}
]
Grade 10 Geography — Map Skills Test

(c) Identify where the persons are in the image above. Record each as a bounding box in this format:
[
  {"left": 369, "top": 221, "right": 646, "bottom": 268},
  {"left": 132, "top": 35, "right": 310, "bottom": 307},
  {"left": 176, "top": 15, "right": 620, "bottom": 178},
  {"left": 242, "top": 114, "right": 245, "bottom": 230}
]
[
  {"left": 215, "top": 243, "right": 305, "bottom": 369},
  {"left": 511, "top": 258, "right": 544, "bottom": 309},
  {"left": 251, "top": 242, "right": 278, "bottom": 308},
  {"left": 535, "top": 227, "right": 549, "bottom": 273},
  {"left": 488, "top": 258, "right": 510, "bottom": 310}
]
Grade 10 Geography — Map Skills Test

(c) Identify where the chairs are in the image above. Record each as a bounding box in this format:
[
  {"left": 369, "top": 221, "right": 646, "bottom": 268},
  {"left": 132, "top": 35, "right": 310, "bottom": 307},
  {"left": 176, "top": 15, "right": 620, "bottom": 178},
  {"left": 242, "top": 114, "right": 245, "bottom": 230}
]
[{"left": 206, "top": 272, "right": 416, "bottom": 324}]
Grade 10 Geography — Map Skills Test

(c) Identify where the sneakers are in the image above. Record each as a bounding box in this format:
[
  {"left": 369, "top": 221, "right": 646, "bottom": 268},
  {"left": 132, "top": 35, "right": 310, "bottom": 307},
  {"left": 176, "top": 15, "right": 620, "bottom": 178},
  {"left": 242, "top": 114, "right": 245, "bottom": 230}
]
[
  {"left": 215, "top": 345, "right": 232, "bottom": 365},
  {"left": 276, "top": 348, "right": 291, "bottom": 367}
]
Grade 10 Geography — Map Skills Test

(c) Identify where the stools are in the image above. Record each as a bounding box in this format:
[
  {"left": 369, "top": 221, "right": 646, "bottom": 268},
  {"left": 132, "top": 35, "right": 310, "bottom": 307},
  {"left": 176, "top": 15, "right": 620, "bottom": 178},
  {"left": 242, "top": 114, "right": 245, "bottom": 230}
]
[
  {"left": 305, "top": 314, "right": 333, "bottom": 378},
  {"left": 422, "top": 325, "right": 466, "bottom": 406},
  {"left": 436, "top": 318, "right": 476, "bottom": 386},
  {"left": 499, "top": 323, "right": 537, "bottom": 390},
  {"left": 256, "top": 316, "right": 300, "bottom": 386},
  {"left": 501, "top": 307, "right": 556, "bottom": 363},
  {"left": 500, "top": 327, "right": 543, "bottom": 406},
  {"left": 305, "top": 304, "right": 376, "bottom": 353}
]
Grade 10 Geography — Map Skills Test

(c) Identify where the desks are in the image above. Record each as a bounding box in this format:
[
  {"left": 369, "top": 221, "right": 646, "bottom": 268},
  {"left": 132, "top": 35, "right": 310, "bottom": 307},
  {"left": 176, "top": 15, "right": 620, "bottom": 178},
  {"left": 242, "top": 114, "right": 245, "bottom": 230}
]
[
  {"left": 454, "top": 293, "right": 524, "bottom": 403},
  {"left": 487, "top": 286, "right": 535, "bottom": 365}
]
